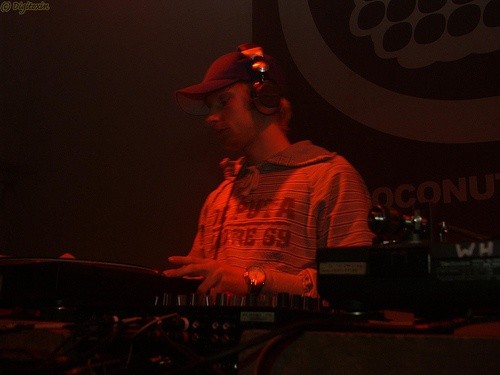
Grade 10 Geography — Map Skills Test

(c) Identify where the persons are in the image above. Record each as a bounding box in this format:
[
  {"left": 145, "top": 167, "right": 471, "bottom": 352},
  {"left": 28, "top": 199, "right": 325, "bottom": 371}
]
[{"left": 58, "top": 48, "right": 375, "bottom": 304}]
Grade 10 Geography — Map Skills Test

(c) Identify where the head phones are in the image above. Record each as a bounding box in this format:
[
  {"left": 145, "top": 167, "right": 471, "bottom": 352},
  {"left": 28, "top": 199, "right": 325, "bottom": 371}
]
[{"left": 238, "top": 42, "right": 282, "bottom": 115}]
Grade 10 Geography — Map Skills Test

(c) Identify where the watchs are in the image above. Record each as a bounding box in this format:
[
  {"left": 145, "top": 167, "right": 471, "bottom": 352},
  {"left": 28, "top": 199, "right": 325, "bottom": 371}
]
[{"left": 242, "top": 265, "right": 267, "bottom": 299}]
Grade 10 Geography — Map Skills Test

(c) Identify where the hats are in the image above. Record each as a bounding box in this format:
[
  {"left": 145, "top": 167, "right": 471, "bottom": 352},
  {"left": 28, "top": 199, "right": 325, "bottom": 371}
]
[{"left": 175, "top": 50, "right": 286, "bottom": 117}]
